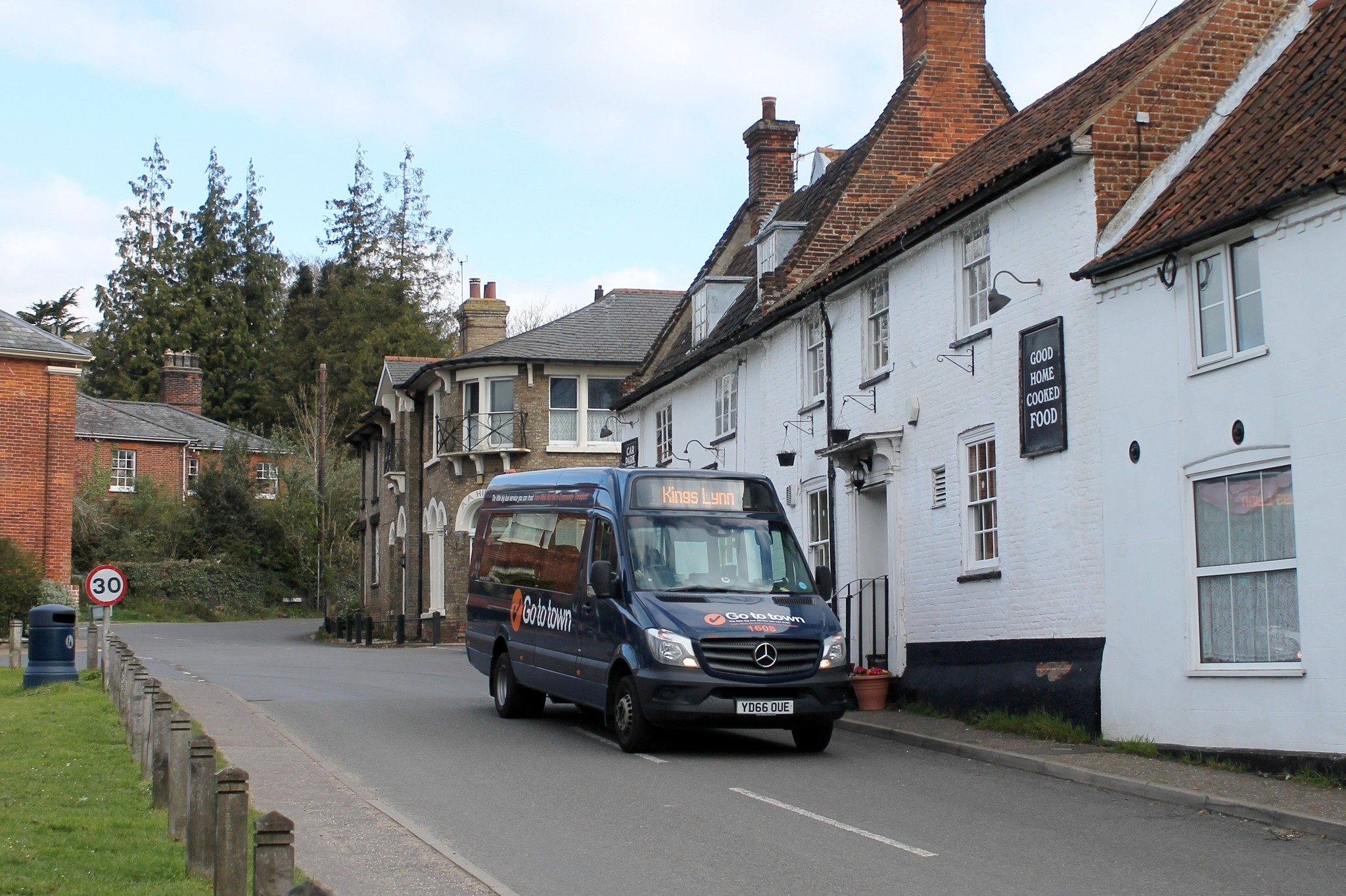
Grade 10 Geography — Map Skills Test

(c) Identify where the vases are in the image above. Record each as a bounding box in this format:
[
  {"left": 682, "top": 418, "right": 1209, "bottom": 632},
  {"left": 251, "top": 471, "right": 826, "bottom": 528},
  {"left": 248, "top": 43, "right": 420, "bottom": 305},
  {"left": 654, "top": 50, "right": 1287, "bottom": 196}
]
[{"left": 850, "top": 673, "right": 889, "bottom": 710}]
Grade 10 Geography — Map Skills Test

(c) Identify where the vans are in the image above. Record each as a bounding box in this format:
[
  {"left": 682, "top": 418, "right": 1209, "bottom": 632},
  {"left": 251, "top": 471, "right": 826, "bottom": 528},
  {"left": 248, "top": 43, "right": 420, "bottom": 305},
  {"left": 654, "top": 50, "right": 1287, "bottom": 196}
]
[{"left": 464, "top": 466, "right": 852, "bottom": 754}]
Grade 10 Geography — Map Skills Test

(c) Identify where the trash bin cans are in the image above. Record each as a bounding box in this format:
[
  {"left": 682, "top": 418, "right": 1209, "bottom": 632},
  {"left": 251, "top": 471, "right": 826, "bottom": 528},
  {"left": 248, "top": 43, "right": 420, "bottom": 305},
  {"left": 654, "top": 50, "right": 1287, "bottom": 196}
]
[{"left": 22, "top": 603, "right": 79, "bottom": 689}]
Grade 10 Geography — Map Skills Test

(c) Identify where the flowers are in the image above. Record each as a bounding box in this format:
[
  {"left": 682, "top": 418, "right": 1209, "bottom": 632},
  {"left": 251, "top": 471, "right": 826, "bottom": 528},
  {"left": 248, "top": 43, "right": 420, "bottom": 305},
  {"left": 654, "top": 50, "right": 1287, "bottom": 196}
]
[{"left": 852, "top": 665, "right": 891, "bottom": 677}]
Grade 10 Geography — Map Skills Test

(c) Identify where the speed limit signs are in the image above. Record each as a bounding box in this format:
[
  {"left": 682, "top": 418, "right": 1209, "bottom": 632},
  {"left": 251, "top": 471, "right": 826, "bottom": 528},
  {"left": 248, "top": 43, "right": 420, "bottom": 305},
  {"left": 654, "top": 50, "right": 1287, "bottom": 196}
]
[{"left": 85, "top": 566, "right": 127, "bottom": 605}]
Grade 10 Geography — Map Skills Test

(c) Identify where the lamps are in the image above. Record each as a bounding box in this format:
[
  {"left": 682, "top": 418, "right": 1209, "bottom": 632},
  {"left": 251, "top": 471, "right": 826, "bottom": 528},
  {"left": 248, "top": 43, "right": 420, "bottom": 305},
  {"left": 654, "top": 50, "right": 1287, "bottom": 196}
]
[
  {"left": 660, "top": 450, "right": 691, "bottom": 464},
  {"left": 987, "top": 271, "right": 1041, "bottom": 316},
  {"left": 845, "top": 453, "right": 873, "bottom": 495},
  {"left": 400, "top": 554, "right": 407, "bottom": 567},
  {"left": 600, "top": 413, "right": 633, "bottom": 438},
  {"left": 684, "top": 440, "right": 717, "bottom": 454}
]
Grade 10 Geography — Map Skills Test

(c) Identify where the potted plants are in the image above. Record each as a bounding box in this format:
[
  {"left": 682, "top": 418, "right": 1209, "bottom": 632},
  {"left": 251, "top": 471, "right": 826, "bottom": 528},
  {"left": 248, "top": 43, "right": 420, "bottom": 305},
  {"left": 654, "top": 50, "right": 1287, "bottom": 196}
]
[
  {"left": 828, "top": 424, "right": 850, "bottom": 444},
  {"left": 777, "top": 448, "right": 796, "bottom": 466}
]
